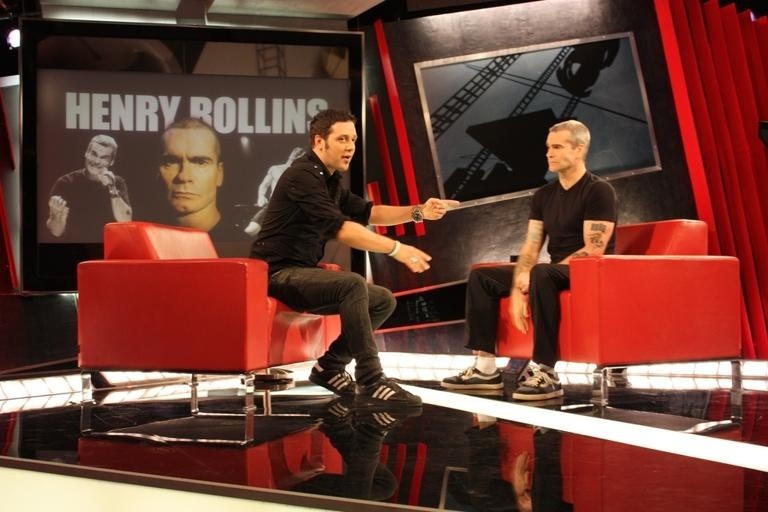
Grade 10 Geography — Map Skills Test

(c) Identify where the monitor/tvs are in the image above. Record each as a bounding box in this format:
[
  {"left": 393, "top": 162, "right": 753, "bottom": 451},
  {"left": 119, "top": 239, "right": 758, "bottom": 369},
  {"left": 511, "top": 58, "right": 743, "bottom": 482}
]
[
  {"left": 20, "top": 16, "right": 366, "bottom": 294},
  {"left": 413, "top": 31, "right": 662, "bottom": 212}
]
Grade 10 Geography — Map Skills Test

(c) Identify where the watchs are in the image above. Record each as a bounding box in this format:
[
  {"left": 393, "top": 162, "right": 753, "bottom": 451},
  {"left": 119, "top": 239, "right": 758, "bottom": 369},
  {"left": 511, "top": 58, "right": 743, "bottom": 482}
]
[{"left": 412, "top": 205, "right": 423, "bottom": 224}]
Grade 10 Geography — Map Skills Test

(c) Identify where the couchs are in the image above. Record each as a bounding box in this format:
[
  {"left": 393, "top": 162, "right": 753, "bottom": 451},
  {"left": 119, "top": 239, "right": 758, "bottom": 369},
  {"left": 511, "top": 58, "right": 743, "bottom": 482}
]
[
  {"left": 493, "top": 419, "right": 743, "bottom": 512},
  {"left": 471, "top": 217, "right": 742, "bottom": 366},
  {"left": 74, "top": 221, "right": 340, "bottom": 446},
  {"left": 76, "top": 424, "right": 344, "bottom": 489}
]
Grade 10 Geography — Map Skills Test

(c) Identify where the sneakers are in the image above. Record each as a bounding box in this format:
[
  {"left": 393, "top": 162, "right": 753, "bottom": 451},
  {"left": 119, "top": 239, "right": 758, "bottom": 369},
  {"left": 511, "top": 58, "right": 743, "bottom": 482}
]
[
  {"left": 512, "top": 368, "right": 564, "bottom": 400},
  {"left": 354, "top": 375, "right": 421, "bottom": 405},
  {"left": 440, "top": 367, "right": 504, "bottom": 390},
  {"left": 352, "top": 396, "right": 423, "bottom": 429},
  {"left": 312, "top": 396, "right": 351, "bottom": 432},
  {"left": 309, "top": 362, "right": 354, "bottom": 396}
]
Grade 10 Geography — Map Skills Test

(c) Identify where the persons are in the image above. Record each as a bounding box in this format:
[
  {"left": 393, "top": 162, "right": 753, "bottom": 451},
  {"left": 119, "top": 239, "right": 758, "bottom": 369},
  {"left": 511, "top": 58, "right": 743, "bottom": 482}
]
[
  {"left": 284, "top": 395, "right": 421, "bottom": 502},
  {"left": 245, "top": 147, "right": 305, "bottom": 237},
  {"left": 239, "top": 108, "right": 460, "bottom": 406},
  {"left": 440, "top": 121, "right": 617, "bottom": 401},
  {"left": 463, "top": 411, "right": 570, "bottom": 510},
  {"left": 46, "top": 135, "right": 132, "bottom": 238},
  {"left": 139, "top": 118, "right": 257, "bottom": 261}
]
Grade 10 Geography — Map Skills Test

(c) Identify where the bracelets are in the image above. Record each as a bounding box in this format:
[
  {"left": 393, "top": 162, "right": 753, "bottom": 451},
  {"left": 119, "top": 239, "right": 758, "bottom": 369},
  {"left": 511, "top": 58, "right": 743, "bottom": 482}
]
[
  {"left": 387, "top": 240, "right": 400, "bottom": 257},
  {"left": 109, "top": 191, "right": 121, "bottom": 198}
]
